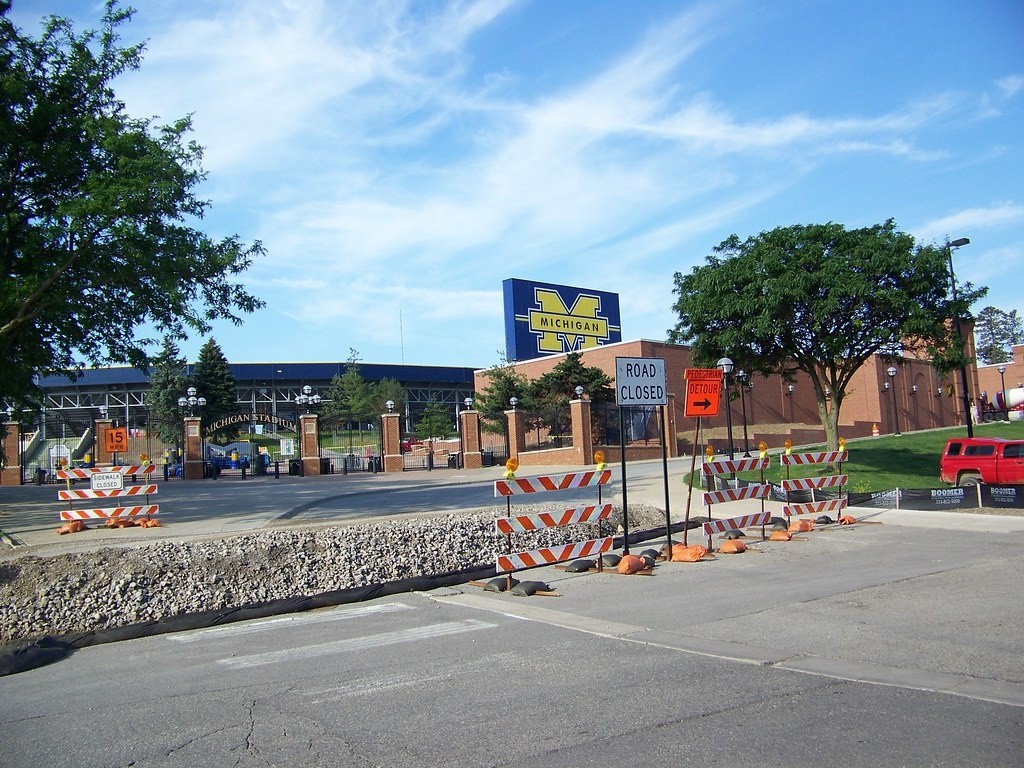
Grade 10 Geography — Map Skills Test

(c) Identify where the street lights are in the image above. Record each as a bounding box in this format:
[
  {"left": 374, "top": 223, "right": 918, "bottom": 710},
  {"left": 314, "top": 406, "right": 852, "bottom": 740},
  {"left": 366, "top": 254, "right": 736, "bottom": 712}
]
[
  {"left": 887, "top": 367, "right": 902, "bottom": 436},
  {"left": 994, "top": 367, "right": 1011, "bottom": 421},
  {"left": 947, "top": 238, "right": 974, "bottom": 438},
  {"left": 715, "top": 356, "right": 735, "bottom": 461},
  {"left": 733, "top": 370, "right": 750, "bottom": 458}
]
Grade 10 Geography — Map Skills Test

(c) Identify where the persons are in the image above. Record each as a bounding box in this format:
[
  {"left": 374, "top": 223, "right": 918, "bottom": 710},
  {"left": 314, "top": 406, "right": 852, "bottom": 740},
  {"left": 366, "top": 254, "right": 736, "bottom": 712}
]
[
  {"left": 970, "top": 401, "right": 978, "bottom": 425},
  {"left": 981, "top": 391, "right": 988, "bottom": 402}
]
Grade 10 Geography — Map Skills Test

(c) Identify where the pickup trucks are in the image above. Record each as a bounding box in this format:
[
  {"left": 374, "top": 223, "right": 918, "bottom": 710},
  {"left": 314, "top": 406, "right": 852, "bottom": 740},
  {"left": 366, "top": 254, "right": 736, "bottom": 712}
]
[
  {"left": 401, "top": 436, "right": 423, "bottom": 454},
  {"left": 938, "top": 437, "right": 1024, "bottom": 489}
]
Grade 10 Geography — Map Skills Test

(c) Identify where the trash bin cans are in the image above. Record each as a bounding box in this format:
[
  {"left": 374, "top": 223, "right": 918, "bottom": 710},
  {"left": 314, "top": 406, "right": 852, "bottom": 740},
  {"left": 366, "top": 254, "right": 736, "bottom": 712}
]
[
  {"left": 205, "top": 461, "right": 211, "bottom": 478},
  {"left": 448, "top": 453, "right": 459, "bottom": 468},
  {"left": 288, "top": 459, "right": 301, "bottom": 474},
  {"left": 483, "top": 451, "right": 493, "bottom": 466},
  {"left": 368, "top": 456, "right": 381, "bottom": 471},
  {"left": 320, "top": 458, "right": 330, "bottom": 473}
]
